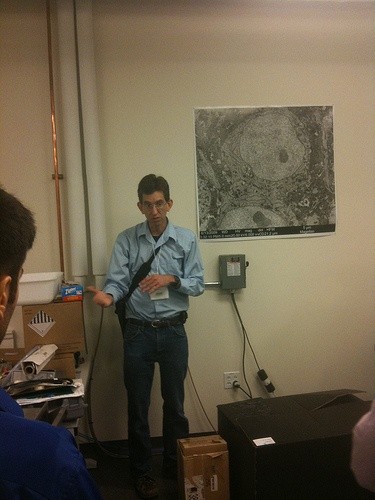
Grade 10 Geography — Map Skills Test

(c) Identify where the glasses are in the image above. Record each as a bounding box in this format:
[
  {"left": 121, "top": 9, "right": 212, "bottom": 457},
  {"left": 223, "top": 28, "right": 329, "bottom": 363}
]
[{"left": 141, "top": 200, "right": 167, "bottom": 209}]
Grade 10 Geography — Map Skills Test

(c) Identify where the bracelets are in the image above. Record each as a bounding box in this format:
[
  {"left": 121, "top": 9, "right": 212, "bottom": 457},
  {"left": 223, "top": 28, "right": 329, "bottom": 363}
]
[{"left": 169, "top": 275, "right": 180, "bottom": 290}]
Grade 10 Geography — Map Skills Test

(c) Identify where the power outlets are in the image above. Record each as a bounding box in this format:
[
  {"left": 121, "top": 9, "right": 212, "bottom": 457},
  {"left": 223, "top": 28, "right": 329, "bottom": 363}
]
[{"left": 223, "top": 371, "right": 240, "bottom": 388}]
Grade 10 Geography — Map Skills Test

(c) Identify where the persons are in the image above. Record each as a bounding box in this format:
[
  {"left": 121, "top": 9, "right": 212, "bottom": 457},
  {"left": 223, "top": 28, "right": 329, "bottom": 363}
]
[
  {"left": 0, "top": 189, "right": 104, "bottom": 499},
  {"left": 84, "top": 174, "right": 206, "bottom": 500}
]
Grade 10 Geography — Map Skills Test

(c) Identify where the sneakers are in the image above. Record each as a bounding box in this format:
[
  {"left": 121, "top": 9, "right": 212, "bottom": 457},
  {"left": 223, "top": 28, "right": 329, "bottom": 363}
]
[
  {"left": 134, "top": 473, "right": 160, "bottom": 499},
  {"left": 160, "top": 457, "right": 177, "bottom": 474}
]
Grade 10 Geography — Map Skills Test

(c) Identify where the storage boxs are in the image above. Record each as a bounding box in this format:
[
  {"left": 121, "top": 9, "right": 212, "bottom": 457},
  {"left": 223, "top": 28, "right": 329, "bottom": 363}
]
[
  {"left": 45, "top": 354, "right": 76, "bottom": 379},
  {"left": 178, "top": 388, "right": 372, "bottom": 500},
  {"left": 15, "top": 301, "right": 85, "bottom": 354}
]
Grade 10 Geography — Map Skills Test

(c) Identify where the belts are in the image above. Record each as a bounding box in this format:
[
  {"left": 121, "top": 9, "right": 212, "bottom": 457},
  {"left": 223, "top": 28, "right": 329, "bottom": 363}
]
[{"left": 128, "top": 318, "right": 181, "bottom": 328}]
